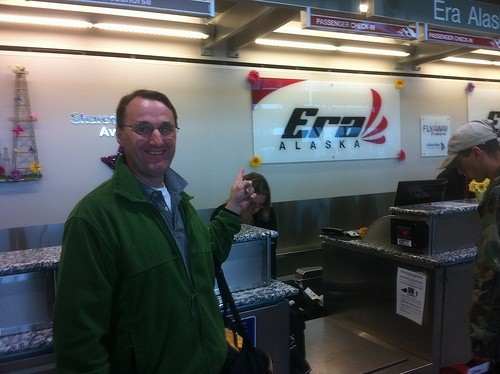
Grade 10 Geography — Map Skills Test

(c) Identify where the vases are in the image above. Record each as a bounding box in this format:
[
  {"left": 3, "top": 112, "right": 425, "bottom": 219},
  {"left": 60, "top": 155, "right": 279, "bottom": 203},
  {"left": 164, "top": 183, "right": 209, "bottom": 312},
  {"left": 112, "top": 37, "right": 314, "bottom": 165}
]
[{"left": 475, "top": 192, "right": 485, "bottom": 204}]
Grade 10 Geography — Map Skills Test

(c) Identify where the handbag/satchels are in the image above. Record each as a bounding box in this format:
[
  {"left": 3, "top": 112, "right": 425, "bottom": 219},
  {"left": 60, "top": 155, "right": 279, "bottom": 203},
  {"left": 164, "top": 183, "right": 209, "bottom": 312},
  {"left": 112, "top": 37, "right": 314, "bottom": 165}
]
[
  {"left": 439, "top": 358, "right": 484, "bottom": 374},
  {"left": 222, "top": 316, "right": 273, "bottom": 374}
]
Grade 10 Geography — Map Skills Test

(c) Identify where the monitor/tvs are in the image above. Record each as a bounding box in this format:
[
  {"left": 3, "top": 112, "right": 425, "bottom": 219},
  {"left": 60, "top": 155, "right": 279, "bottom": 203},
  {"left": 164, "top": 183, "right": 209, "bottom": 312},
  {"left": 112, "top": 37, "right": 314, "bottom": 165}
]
[{"left": 394, "top": 179, "right": 447, "bottom": 206}]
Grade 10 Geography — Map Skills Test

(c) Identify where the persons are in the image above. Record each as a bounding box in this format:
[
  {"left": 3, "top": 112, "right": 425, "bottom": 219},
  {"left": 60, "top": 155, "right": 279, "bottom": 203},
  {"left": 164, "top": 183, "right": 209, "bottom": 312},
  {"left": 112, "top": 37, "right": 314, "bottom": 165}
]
[
  {"left": 436, "top": 119, "right": 499, "bottom": 373},
  {"left": 209, "top": 171, "right": 311, "bottom": 373},
  {"left": 50, "top": 88, "right": 259, "bottom": 373}
]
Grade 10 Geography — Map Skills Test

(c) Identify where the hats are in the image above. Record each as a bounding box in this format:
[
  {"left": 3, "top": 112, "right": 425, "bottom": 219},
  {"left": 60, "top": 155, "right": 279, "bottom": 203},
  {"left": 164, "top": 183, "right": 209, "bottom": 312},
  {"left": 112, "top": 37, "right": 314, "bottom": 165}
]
[{"left": 440, "top": 118, "right": 497, "bottom": 169}]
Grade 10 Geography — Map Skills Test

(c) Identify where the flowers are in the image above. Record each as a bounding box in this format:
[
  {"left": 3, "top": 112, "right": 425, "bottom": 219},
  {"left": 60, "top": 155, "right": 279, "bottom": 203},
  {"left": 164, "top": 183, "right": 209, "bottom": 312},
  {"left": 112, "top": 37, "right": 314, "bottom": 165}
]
[
  {"left": 248, "top": 70, "right": 261, "bottom": 84},
  {"left": 394, "top": 79, "right": 407, "bottom": 89},
  {"left": 250, "top": 155, "right": 261, "bottom": 168},
  {"left": 469, "top": 178, "right": 491, "bottom": 193},
  {"left": 398, "top": 150, "right": 406, "bottom": 160},
  {"left": 9, "top": 169, "right": 23, "bottom": 180},
  {"left": 466, "top": 82, "right": 476, "bottom": 93},
  {"left": 29, "top": 162, "right": 41, "bottom": 173}
]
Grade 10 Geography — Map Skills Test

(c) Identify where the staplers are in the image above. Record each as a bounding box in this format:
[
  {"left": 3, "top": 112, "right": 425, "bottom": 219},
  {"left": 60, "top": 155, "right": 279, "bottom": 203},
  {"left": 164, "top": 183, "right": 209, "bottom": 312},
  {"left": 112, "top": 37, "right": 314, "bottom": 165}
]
[{"left": 320, "top": 227, "right": 345, "bottom": 237}]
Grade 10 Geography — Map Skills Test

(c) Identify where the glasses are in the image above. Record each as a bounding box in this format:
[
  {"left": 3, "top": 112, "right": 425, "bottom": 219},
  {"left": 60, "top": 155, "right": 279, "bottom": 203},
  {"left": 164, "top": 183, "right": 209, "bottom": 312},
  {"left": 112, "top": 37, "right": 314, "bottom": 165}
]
[
  {"left": 249, "top": 201, "right": 265, "bottom": 208},
  {"left": 123, "top": 121, "right": 180, "bottom": 135}
]
